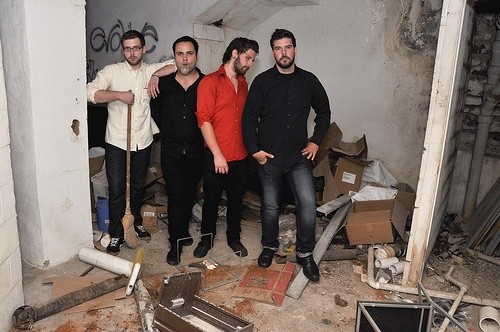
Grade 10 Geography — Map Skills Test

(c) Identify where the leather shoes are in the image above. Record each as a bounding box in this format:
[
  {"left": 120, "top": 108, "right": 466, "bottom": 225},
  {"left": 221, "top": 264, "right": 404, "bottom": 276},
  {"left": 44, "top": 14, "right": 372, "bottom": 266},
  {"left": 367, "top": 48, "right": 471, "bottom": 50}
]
[
  {"left": 295, "top": 254, "right": 321, "bottom": 280},
  {"left": 259, "top": 246, "right": 275, "bottom": 268}
]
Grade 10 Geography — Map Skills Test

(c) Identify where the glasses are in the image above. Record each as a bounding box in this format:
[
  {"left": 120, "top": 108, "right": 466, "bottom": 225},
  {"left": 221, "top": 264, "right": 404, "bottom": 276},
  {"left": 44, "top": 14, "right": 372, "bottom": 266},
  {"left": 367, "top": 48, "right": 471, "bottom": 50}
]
[{"left": 124, "top": 46, "right": 144, "bottom": 52}]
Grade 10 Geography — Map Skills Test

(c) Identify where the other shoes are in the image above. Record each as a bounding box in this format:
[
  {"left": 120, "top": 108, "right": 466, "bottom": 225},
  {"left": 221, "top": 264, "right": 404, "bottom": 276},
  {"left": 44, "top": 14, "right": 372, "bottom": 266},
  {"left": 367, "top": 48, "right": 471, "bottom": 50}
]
[
  {"left": 194, "top": 240, "right": 213, "bottom": 257},
  {"left": 183, "top": 230, "right": 193, "bottom": 246},
  {"left": 229, "top": 239, "right": 247, "bottom": 256},
  {"left": 167, "top": 247, "right": 181, "bottom": 264}
]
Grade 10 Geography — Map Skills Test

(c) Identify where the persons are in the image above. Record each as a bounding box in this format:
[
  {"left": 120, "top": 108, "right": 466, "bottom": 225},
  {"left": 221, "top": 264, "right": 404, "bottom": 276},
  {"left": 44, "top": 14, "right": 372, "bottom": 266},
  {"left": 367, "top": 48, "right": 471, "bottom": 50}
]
[
  {"left": 192, "top": 37, "right": 260, "bottom": 258},
  {"left": 87, "top": 29, "right": 178, "bottom": 254},
  {"left": 149, "top": 35, "right": 211, "bottom": 265},
  {"left": 242, "top": 28, "right": 332, "bottom": 283}
]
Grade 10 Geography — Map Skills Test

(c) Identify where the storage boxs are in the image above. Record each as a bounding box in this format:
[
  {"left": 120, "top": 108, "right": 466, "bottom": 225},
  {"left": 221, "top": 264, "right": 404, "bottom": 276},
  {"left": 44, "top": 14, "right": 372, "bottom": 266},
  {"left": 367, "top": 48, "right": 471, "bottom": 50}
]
[
  {"left": 153, "top": 272, "right": 254, "bottom": 332},
  {"left": 141, "top": 208, "right": 158, "bottom": 229},
  {"left": 333, "top": 157, "right": 415, "bottom": 245},
  {"left": 91, "top": 168, "right": 109, "bottom": 204},
  {"left": 145, "top": 164, "right": 163, "bottom": 186}
]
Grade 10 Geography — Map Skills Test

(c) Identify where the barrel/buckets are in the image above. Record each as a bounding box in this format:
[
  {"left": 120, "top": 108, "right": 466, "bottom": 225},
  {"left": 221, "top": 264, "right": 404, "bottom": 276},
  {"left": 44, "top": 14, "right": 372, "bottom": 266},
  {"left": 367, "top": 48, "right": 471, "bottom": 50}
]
[{"left": 94, "top": 200, "right": 109, "bottom": 233}]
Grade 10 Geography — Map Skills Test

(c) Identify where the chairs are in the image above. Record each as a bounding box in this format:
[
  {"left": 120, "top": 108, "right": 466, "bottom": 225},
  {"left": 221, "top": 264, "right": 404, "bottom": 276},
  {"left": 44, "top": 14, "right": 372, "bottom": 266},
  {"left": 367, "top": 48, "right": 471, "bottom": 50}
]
[{"left": 356, "top": 279, "right": 469, "bottom": 332}]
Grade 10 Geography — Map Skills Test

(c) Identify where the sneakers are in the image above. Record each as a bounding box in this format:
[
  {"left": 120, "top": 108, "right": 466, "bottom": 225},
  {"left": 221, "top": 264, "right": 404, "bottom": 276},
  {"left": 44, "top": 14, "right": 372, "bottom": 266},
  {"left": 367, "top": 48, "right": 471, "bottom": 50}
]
[
  {"left": 106, "top": 237, "right": 126, "bottom": 255},
  {"left": 134, "top": 225, "right": 150, "bottom": 241}
]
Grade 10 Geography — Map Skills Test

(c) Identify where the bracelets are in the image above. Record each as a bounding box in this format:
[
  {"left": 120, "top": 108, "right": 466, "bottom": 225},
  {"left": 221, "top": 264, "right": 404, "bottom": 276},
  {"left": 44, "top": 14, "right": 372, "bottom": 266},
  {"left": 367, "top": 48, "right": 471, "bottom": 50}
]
[{"left": 151, "top": 75, "right": 160, "bottom": 78}]
